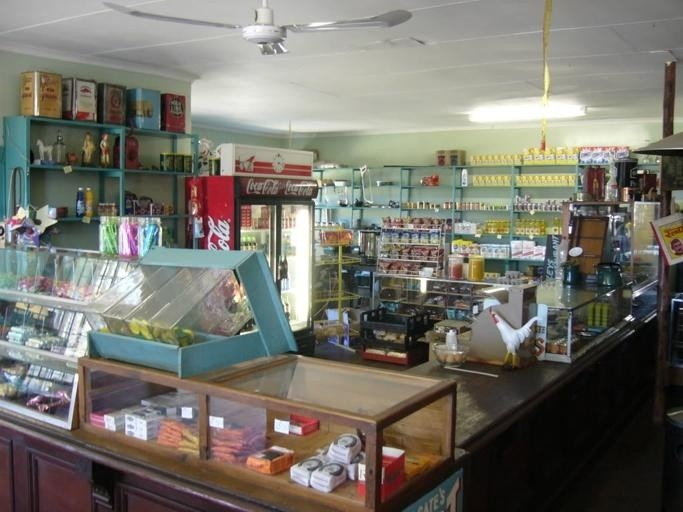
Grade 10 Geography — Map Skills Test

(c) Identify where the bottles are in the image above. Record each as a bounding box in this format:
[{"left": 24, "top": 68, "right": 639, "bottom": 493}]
[
  {"left": 188, "top": 185, "right": 201, "bottom": 226},
  {"left": 484, "top": 216, "right": 562, "bottom": 234},
  {"left": 51, "top": 128, "right": 66, "bottom": 164},
  {"left": 605, "top": 174, "right": 619, "bottom": 201},
  {"left": 333, "top": 180, "right": 348, "bottom": 206},
  {"left": 96, "top": 203, "right": 116, "bottom": 217},
  {"left": 142, "top": 217, "right": 162, "bottom": 259},
  {"left": 97, "top": 216, "right": 119, "bottom": 254},
  {"left": 445, "top": 329, "right": 457, "bottom": 352},
  {"left": 75, "top": 188, "right": 85, "bottom": 217},
  {"left": 315, "top": 220, "right": 353, "bottom": 244},
  {"left": 592, "top": 176, "right": 600, "bottom": 200},
  {"left": 446, "top": 253, "right": 463, "bottom": 281},
  {"left": 467, "top": 253, "right": 484, "bottom": 281},
  {"left": 99, "top": 133, "right": 111, "bottom": 167},
  {"left": 117, "top": 216, "right": 143, "bottom": 259},
  {"left": 84, "top": 188, "right": 93, "bottom": 217},
  {"left": 240, "top": 237, "right": 290, "bottom": 331}
]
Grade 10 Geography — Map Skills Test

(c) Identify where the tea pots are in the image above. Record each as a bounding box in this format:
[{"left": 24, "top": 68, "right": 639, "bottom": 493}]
[
  {"left": 592, "top": 261, "right": 623, "bottom": 286},
  {"left": 559, "top": 260, "right": 582, "bottom": 283}
]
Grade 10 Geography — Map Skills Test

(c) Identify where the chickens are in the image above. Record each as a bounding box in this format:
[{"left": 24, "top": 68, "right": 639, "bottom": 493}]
[{"left": 488, "top": 306, "right": 541, "bottom": 370}]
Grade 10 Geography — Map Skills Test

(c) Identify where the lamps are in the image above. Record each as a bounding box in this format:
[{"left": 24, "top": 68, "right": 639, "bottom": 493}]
[{"left": 469, "top": 106, "right": 587, "bottom": 123}]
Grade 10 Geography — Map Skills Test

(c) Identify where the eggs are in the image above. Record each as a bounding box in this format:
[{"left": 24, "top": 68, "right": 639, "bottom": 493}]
[{"left": 438, "top": 354, "right": 464, "bottom": 364}]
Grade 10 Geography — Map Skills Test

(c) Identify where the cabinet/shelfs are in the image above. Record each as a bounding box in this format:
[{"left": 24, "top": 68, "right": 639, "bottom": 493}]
[
  {"left": 78, "top": 349, "right": 457, "bottom": 512},
  {"left": 0, "top": 242, "right": 144, "bottom": 431},
  {"left": 5, "top": 115, "right": 199, "bottom": 249}
]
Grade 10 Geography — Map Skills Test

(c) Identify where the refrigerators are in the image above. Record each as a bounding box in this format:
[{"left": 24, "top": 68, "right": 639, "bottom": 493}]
[{"left": 184, "top": 175, "right": 319, "bottom": 357}]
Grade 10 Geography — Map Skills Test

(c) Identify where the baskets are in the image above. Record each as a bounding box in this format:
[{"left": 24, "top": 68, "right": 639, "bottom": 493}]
[{"left": 359, "top": 307, "right": 429, "bottom": 367}]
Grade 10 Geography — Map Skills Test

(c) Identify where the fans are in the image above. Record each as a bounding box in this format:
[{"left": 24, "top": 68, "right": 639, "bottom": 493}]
[{"left": 103, "top": 0, "right": 412, "bottom": 55}]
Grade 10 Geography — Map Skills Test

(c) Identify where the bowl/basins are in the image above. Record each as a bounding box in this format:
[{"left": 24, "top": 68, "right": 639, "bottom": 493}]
[{"left": 432, "top": 342, "right": 471, "bottom": 368}]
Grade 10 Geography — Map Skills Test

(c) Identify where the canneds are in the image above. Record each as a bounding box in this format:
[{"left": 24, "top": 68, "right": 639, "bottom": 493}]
[
  {"left": 208, "top": 157, "right": 220, "bottom": 176},
  {"left": 402, "top": 201, "right": 456, "bottom": 209},
  {"left": 160, "top": 152, "right": 193, "bottom": 172},
  {"left": 546, "top": 336, "right": 581, "bottom": 355},
  {"left": 623, "top": 187, "right": 634, "bottom": 203},
  {"left": 258, "top": 207, "right": 296, "bottom": 229}
]
